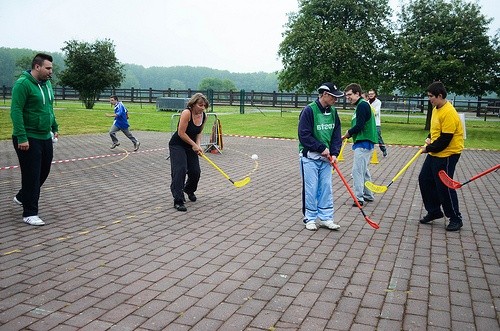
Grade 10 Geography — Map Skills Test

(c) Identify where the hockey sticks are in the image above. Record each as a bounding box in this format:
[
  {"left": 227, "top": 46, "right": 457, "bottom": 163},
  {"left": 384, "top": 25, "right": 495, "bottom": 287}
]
[
  {"left": 436, "top": 163, "right": 500, "bottom": 190},
  {"left": 198, "top": 152, "right": 251, "bottom": 188},
  {"left": 328, "top": 154, "right": 379, "bottom": 228},
  {"left": 364, "top": 140, "right": 429, "bottom": 193},
  {"left": 332, "top": 139, "right": 350, "bottom": 174}
]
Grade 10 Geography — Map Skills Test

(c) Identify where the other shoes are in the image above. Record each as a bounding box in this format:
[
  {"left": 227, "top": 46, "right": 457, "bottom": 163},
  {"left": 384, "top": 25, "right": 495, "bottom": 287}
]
[
  {"left": 353, "top": 201, "right": 363, "bottom": 206},
  {"left": 383, "top": 150, "right": 387, "bottom": 156},
  {"left": 364, "top": 198, "right": 373, "bottom": 201},
  {"left": 134, "top": 142, "right": 140, "bottom": 151},
  {"left": 111, "top": 142, "right": 120, "bottom": 149}
]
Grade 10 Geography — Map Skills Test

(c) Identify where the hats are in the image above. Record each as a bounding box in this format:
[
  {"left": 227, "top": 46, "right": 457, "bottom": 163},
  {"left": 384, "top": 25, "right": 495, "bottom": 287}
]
[{"left": 317, "top": 82, "right": 345, "bottom": 98}]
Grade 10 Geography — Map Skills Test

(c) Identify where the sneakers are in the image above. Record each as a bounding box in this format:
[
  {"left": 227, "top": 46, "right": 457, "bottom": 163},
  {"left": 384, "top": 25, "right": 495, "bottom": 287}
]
[
  {"left": 184, "top": 190, "right": 196, "bottom": 201},
  {"left": 420, "top": 211, "right": 443, "bottom": 223},
  {"left": 447, "top": 218, "right": 463, "bottom": 231},
  {"left": 13, "top": 197, "right": 23, "bottom": 207},
  {"left": 319, "top": 220, "right": 340, "bottom": 230},
  {"left": 23, "top": 215, "right": 45, "bottom": 226},
  {"left": 174, "top": 201, "right": 187, "bottom": 211},
  {"left": 302, "top": 219, "right": 317, "bottom": 230}
]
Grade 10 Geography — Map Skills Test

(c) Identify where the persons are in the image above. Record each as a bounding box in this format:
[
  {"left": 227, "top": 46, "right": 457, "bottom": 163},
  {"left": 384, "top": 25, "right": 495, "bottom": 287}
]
[
  {"left": 9, "top": 54, "right": 60, "bottom": 226},
  {"left": 341, "top": 83, "right": 378, "bottom": 207},
  {"left": 297, "top": 83, "right": 343, "bottom": 229},
  {"left": 418, "top": 82, "right": 464, "bottom": 231},
  {"left": 168, "top": 93, "right": 210, "bottom": 211},
  {"left": 367, "top": 89, "right": 388, "bottom": 157},
  {"left": 105, "top": 95, "right": 140, "bottom": 151}
]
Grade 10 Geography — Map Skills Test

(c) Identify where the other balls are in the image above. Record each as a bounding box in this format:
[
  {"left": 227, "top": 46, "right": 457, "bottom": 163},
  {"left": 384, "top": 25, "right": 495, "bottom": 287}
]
[{"left": 251, "top": 153, "right": 258, "bottom": 160}]
